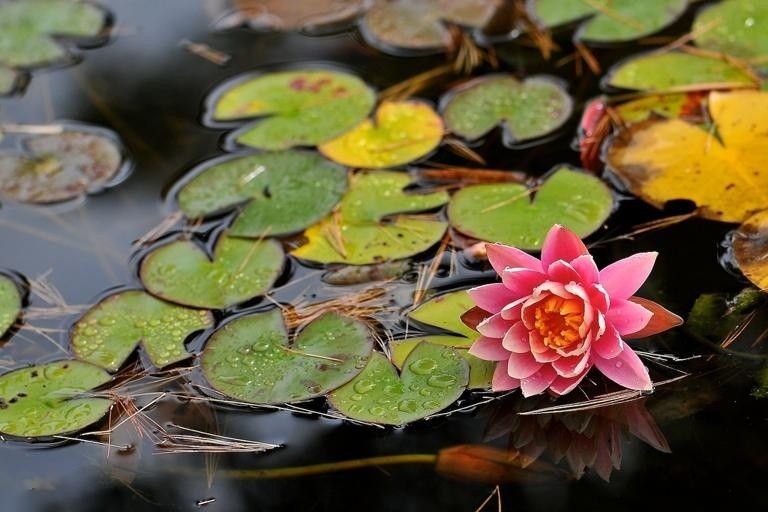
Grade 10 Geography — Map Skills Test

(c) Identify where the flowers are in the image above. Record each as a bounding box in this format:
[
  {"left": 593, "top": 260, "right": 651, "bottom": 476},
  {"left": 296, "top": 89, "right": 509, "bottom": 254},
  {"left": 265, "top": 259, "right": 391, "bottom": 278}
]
[
  {"left": 459, "top": 228, "right": 685, "bottom": 399},
  {"left": 477, "top": 381, "right": 678, "bottom": 485}
]
